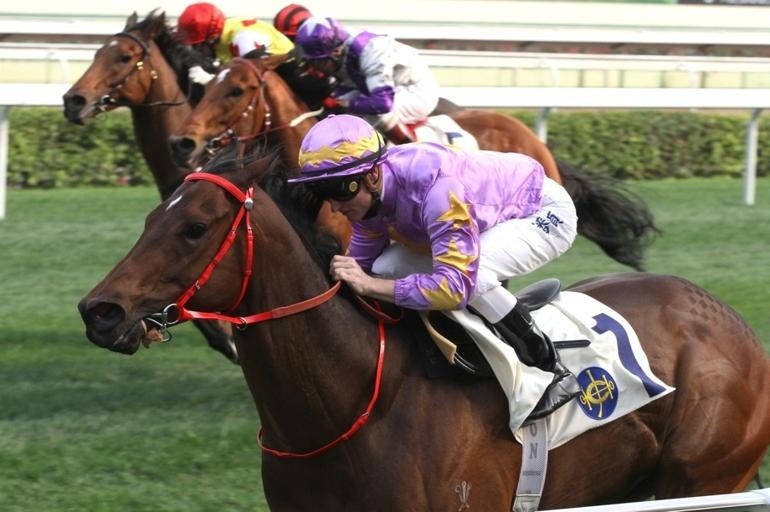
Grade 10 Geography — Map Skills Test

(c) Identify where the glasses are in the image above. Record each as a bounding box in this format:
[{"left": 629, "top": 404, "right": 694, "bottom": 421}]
[{"left": 308, "top": 177, "right": 363, "bottom": 201}]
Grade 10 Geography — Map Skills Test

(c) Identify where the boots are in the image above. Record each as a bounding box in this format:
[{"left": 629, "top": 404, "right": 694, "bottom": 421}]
[{"left": 491, "top": 300, "right": 582, "bottom": 427}]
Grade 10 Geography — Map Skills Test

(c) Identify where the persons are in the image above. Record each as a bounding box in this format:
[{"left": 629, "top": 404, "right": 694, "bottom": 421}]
[
  {"left": 169, "top": 3, "right": 297, "bottom": 105},
  {"left": 286, "top": 114, "right": 583, "bottom": 429},
  {"left": 259, "top": 3, "right": 342, "bottom": 77},
  {"left": 294, "top": 14, "right": 440, "bottom": 146}
]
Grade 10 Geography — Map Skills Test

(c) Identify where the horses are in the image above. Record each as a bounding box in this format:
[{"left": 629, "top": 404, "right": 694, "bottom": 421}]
[
  {"left": 75, "top": 132, "right": 770, "bottom": 512},
  {"left": 63, "top": 8, "right": 664, "bottom": 365}
]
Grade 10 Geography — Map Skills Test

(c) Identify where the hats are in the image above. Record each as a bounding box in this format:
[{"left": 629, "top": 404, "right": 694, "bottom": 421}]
[
  {"left": 273, "top": 4, "right": 347, "bottom": 59},
  {"left": 170, "top": 3, "right": 226, "bottom": 45},
  {"left": 287, "top": 114, "right": 388, "bottom": 183}
]
